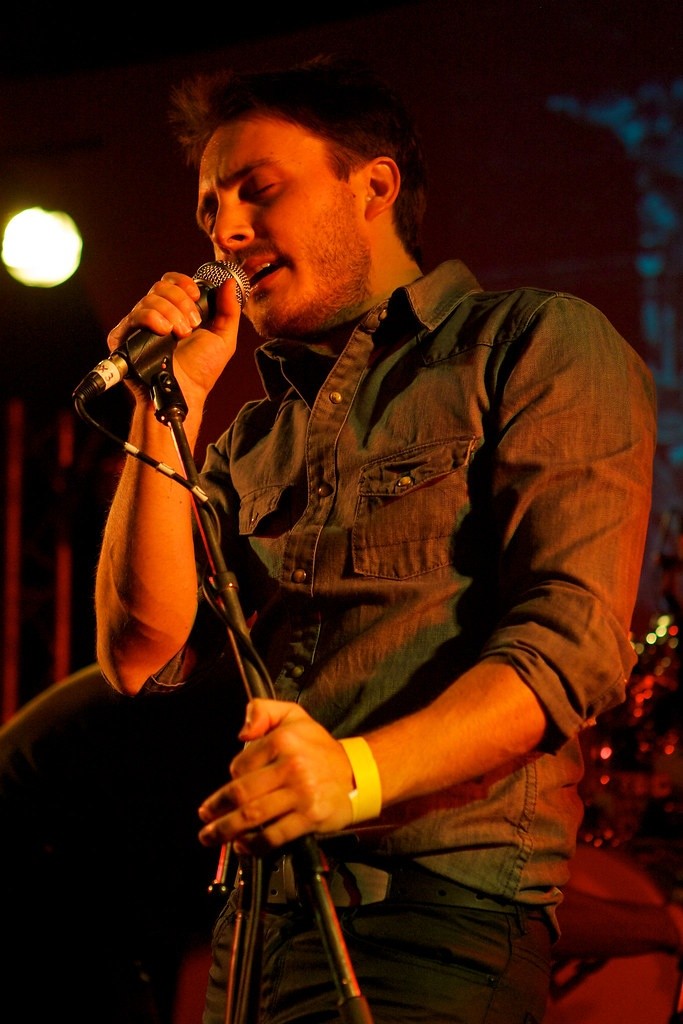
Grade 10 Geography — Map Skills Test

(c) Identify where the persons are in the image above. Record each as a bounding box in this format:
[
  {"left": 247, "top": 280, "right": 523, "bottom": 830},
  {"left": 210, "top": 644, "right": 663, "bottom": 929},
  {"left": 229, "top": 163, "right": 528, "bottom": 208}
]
[{"left": 0, "top": 54, "right": 655, "bottom": 1024}]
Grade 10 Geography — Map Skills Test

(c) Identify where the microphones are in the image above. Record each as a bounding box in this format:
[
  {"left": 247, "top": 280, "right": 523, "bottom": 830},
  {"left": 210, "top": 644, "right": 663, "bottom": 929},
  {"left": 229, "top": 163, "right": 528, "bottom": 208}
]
[{"left": 73, "top": 260, "right": 250, "bottom": 404}]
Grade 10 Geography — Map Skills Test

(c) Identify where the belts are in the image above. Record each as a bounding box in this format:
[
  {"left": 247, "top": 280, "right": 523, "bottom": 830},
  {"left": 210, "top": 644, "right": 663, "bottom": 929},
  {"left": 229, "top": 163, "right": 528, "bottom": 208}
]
[{"left": 231, "top": 851, "right": 547, "bottom": 921}]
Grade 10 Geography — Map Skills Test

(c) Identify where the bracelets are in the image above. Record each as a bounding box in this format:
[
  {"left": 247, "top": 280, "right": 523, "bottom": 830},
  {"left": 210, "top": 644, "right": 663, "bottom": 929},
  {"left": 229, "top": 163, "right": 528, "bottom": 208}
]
[{"left": 335, "top": 735, "right": 382, "bottom": 826}]
